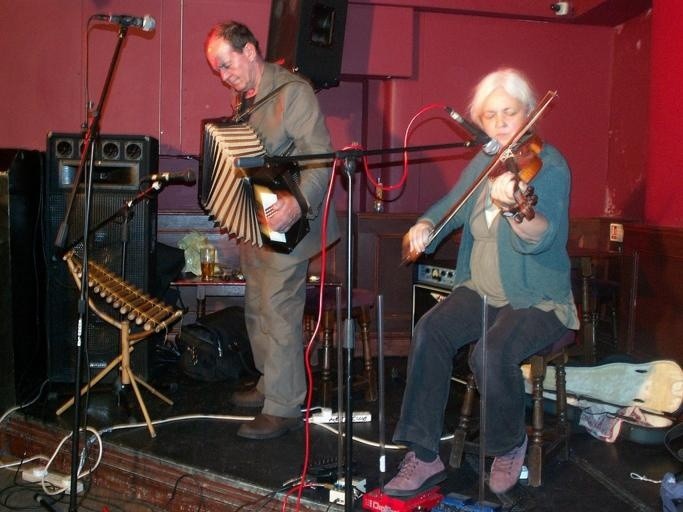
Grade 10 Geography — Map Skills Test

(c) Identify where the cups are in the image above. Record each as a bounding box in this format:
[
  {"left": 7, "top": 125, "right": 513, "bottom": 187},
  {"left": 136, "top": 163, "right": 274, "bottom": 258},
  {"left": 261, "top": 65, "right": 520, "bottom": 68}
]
[{"left": 199, "top": 248, "right": 216, "bottom": 282}]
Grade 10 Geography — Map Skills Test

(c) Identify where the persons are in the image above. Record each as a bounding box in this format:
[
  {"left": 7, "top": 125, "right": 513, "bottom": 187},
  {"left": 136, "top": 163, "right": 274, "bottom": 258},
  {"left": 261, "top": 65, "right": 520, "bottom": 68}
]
[
  {"left": 379, "top": 67, "right": 582, "bottom": 499},
  {"left": 203, "top": 18, "right": 334, "bottom": 443}
]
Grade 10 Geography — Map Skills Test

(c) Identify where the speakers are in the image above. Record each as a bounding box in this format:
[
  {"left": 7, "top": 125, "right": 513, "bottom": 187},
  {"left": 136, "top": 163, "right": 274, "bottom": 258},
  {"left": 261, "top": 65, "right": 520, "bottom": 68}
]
[
  {"left": 45, "top": 131, "right": 159, "bottom": 385},
  {"left": 410, "top": 283, "right": 483, "bottom": 440},
  {"left": 265, "top": 0, "right": 348, "bottom": 90}
]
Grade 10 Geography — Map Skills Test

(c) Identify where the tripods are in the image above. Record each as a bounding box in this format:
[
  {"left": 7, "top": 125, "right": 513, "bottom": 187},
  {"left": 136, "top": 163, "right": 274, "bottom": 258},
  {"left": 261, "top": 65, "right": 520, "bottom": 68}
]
[{"left": 33, "top": 27, "right": 164, "bottom": 512}]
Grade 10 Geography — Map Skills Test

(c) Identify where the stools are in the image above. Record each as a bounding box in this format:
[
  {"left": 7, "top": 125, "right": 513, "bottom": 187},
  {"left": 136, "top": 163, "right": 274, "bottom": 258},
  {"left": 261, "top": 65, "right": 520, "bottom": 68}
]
[
  {"left": 307, "top": 289, "right": 381, "bottom": 405},
  {"left": 448, "top": 343, "right": 574, "bottom": 489}
]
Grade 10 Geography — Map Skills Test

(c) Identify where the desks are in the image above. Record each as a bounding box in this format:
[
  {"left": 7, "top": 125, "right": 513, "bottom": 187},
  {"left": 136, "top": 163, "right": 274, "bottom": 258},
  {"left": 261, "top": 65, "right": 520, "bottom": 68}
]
[{"left": 171, "top": 275, "right": 343, "bottom": 317}]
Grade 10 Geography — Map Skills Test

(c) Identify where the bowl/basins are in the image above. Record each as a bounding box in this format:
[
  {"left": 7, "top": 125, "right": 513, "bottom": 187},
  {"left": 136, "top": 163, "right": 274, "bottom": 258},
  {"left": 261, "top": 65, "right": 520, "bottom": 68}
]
[{"left": 620, "top": 416, "right": 673, "bottom": 446}]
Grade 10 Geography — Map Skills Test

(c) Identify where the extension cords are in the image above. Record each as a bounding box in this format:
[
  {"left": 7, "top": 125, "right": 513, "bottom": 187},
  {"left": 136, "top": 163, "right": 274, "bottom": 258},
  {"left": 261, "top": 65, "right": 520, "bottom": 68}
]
[
  {"left": 312, "top": 411, "right": 372, "bottom": 424},
  {"left": 22, "top": 467, "right": 84, "bottom": 495}
]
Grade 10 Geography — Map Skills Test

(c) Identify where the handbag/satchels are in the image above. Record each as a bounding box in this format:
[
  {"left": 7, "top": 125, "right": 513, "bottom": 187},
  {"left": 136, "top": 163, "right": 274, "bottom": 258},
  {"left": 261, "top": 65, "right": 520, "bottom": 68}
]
[{"left": 181, "top": 306, "right": 261, "bottom": 387}]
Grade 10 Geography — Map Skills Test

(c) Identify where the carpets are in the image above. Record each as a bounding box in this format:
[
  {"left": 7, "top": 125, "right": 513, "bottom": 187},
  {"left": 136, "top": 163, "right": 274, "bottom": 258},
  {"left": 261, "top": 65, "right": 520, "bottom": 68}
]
[{"left": 1, "top": 408, "right": 342, "bottom": 511}]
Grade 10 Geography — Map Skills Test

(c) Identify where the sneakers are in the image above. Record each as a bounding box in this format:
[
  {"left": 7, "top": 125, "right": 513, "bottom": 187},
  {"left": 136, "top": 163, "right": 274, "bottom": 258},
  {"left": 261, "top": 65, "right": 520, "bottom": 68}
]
[
  {"left": 488, "top": 433, "right": 528, "bottom": 494},
  {"left": 383, "top": 451, "right": 449, "bottom": 497},
  {"left": 236, "top": 416, "right": 305, "bottom": 439},
  {"left": 231, "top": 388, "right": 265, "bottom": 408}
]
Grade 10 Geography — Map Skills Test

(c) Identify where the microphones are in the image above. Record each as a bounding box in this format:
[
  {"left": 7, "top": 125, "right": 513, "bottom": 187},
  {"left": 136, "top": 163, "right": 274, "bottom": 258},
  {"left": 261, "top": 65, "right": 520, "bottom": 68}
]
[
  {"left": 449, "top": 110, "right": 499, "bottom": 155},
  {"left": 109, "top": 14, "right": 156, "bottom": 32},
  {"left": 140, "top": 168, "right": 196, "bottom": 187}
]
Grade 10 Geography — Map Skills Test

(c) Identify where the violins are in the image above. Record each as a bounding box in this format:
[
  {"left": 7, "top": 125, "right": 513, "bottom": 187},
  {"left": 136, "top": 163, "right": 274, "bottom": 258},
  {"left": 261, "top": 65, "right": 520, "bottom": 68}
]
[{"left": 488, "top": 134, "right": 542, "bottom": 223}]
